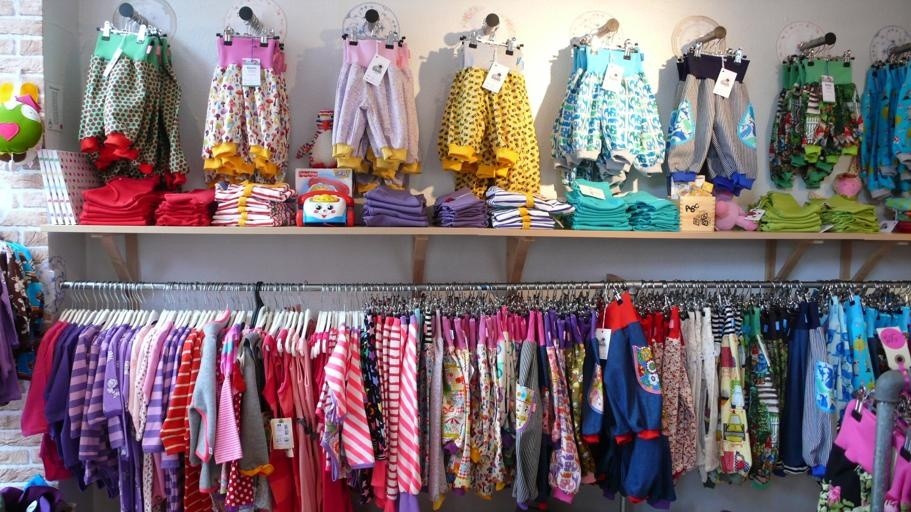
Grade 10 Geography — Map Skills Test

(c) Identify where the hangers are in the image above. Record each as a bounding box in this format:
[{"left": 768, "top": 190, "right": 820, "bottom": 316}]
[{"left": 848, "top": 361, "right": 911, "bottom": 467}]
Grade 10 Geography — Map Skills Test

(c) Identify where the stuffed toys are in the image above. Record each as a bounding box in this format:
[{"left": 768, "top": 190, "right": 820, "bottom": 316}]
[
  {"left": 296, "top": 110, "right": 337, "bottom": 168},
  {"left": 832, "top": 172, "right": 863, "bottom": 198},
  {"left": 294, "top": 189, "right": 355, "bottom": 226},
  {"left": 714, "top": 189, "right": 759, "bottom": 233},
  {"left": 0, "top": 81, "right": 44, "bottom": 162}
]
